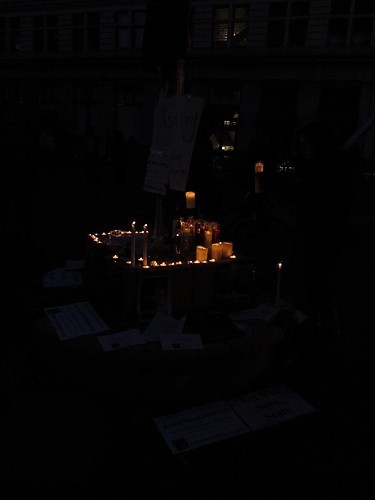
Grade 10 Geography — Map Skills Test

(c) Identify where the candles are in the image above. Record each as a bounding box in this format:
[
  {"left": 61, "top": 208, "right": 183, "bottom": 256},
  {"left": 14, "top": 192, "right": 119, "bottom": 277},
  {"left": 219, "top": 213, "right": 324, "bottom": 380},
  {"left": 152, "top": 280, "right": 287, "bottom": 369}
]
[
  {"left": 181, "top": 189, "right": 233, "bottom": 264},
  {"left": 129, "top": 219, "right": 137, "bottom": 266},
  {"left": 141, "top": 223, "right": 149, "bottom": 268}
]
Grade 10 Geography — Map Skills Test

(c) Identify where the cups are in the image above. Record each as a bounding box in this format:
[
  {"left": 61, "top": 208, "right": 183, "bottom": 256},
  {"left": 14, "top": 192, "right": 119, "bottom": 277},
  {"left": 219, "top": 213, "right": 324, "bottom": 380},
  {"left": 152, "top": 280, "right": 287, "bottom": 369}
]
[
  {"left": 172, "top": 216, "right": 233, "bottom": 262},
  {"left": 185, "top": 193, "right": 196, "bottom": 209}
]
